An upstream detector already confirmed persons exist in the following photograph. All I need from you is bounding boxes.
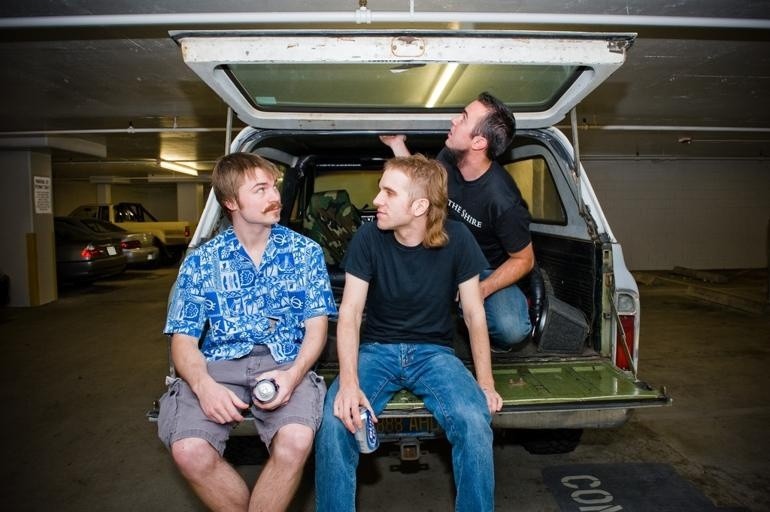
[314,153,503,512]
[380,92,535,353]
[156,152,339,512]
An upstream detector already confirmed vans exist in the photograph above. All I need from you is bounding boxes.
[141,20,676,461]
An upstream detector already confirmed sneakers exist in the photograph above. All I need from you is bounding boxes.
[490,345,514,354]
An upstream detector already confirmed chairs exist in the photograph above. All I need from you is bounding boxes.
[301,189,362,268]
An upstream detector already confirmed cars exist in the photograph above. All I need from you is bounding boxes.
[53,215,130,290]
[75,215,159,266]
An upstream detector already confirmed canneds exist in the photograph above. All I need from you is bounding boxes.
[350,405,381,455]
[250,378,279,403]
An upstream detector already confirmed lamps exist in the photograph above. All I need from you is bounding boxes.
[156,160,198,178]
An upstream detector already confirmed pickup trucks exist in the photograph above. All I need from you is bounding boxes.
[68,201,193,259]
